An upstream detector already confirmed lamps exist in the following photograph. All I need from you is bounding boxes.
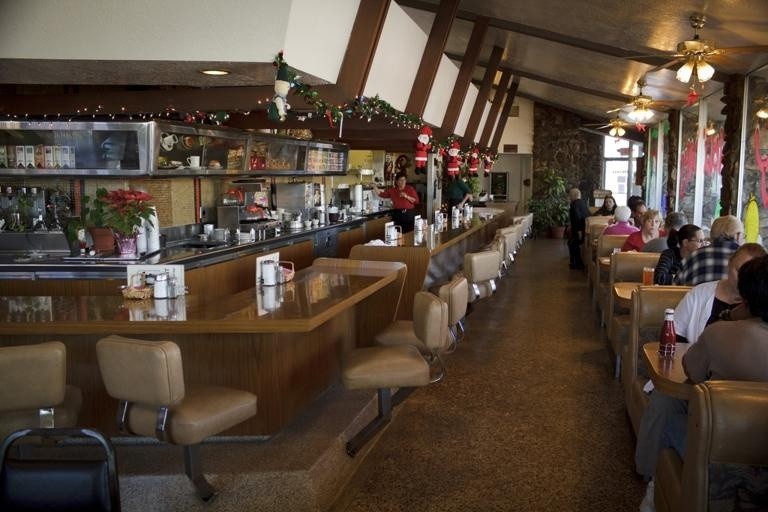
[627,109,655,121]
[676,59,715,83]
[609,127,626,137]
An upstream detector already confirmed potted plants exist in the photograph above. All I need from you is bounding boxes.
[542,173,571,239]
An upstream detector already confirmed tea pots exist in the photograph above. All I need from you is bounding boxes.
[223,188,242,206]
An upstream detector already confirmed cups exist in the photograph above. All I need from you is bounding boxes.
[163,134,178,146]
[305,220,311,227]
[185,135,194,147]
[312,219,319,225]
[186,154,200,169]
[329,207,338,224]
[135,205,159,254]
[642,265,655,285]
[613,247,621,253]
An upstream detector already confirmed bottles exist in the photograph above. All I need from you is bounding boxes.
[262,260,275,287]
[383,203,474,247]
[235,228,241,246]
[154,273,168,298]
[224,228,230,241]
[249,227,256,243]
[276,266,285,283]
[168,278,177,297]
[658,307,677,359]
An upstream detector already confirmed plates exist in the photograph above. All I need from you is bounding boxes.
[159,131,174,151]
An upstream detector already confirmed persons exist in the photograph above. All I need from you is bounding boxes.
[621,208,666,254]
[652,223,707,286]
[631,200,647,230]
[594,195,618,215]
[372,171,421,234]
[566,187,592,271]
[671,214,747,287]
[602,205,637,235]
[443,175,470,218]
[640,209,690,254]
[678,257,767,386]
[627,194,640,203]
[634,242,767,512]
[459,175,472,204]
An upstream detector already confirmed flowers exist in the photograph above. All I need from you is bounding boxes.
[100,189,156,234]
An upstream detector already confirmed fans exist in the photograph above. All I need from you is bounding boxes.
[606,79,688,114]
[623,14,768,73]
[583,115,647,129]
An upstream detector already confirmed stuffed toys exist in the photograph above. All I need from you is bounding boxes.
[466,146,482,179]
[446,141,461,181]
[266,65,294,123]
[482,150,496,178]
[413,124,434,175]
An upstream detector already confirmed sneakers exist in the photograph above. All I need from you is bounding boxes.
[638,480,655,512]
